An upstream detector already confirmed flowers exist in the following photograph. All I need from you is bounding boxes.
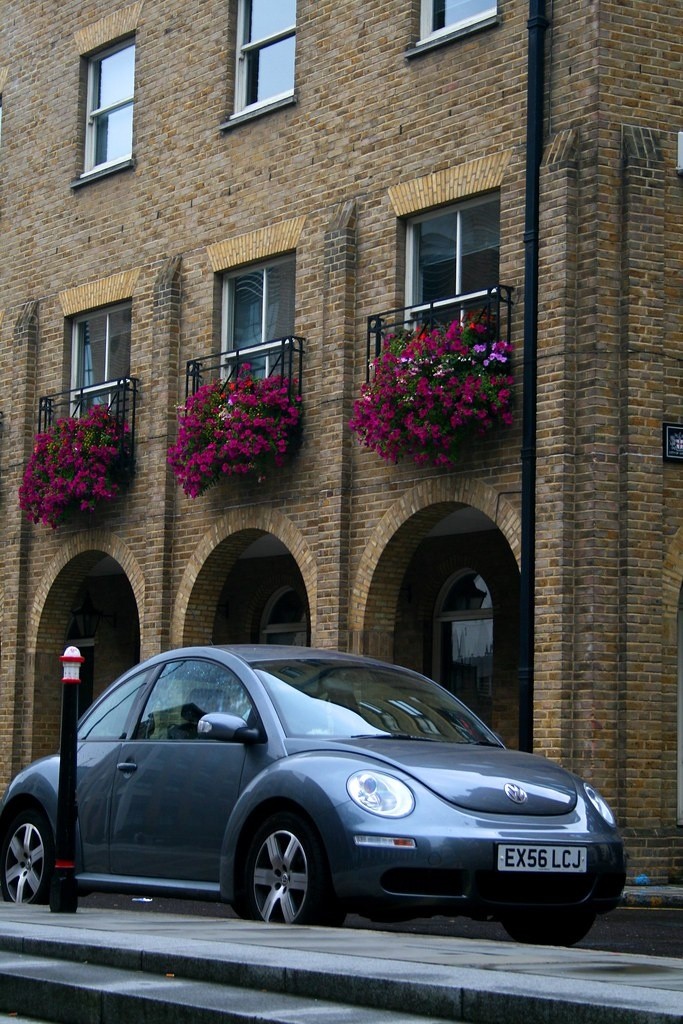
[165,360,303,498]
[346,306,516,467]
[17,402,132,533]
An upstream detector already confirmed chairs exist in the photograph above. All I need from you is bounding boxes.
[314,689,368,734]
[169,687,231,738]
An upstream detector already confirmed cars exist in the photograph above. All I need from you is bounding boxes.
[0,643,628,948]
